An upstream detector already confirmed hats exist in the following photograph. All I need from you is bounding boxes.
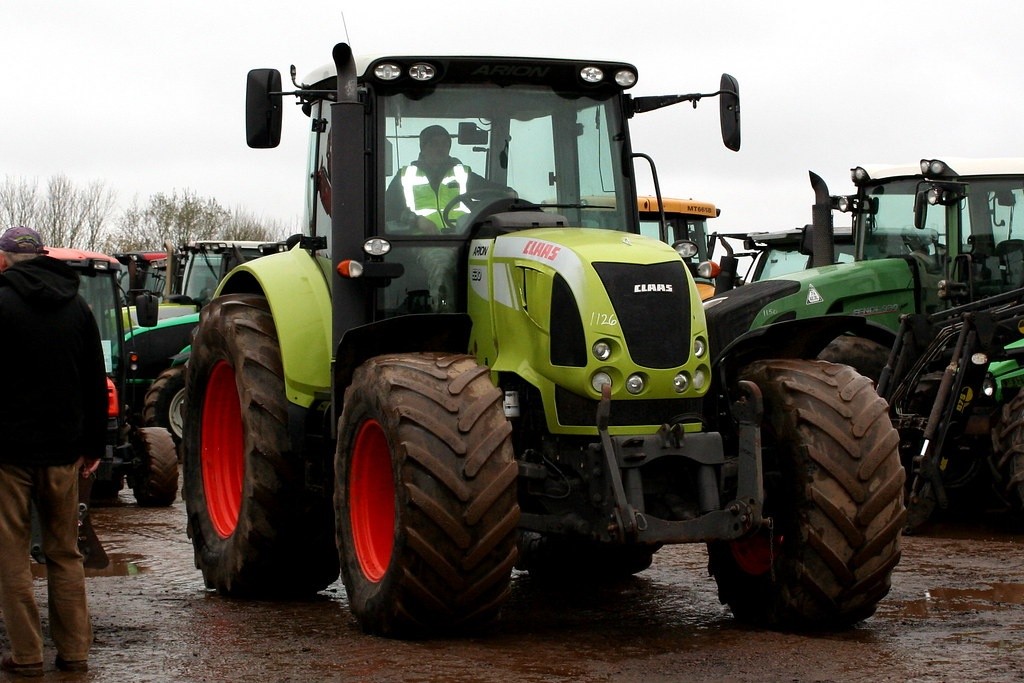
[0,226,49,254]
[420,125,452,150]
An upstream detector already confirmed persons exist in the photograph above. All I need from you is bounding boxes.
[384,125,520,236]
[0,227,109,676]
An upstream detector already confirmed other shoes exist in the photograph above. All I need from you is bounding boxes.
[0,654,43,676]
[55,653,88,673]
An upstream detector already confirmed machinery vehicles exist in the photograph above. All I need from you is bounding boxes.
[38,239,295,509]
[169,41,906,630]
[548,159,1024,518]
